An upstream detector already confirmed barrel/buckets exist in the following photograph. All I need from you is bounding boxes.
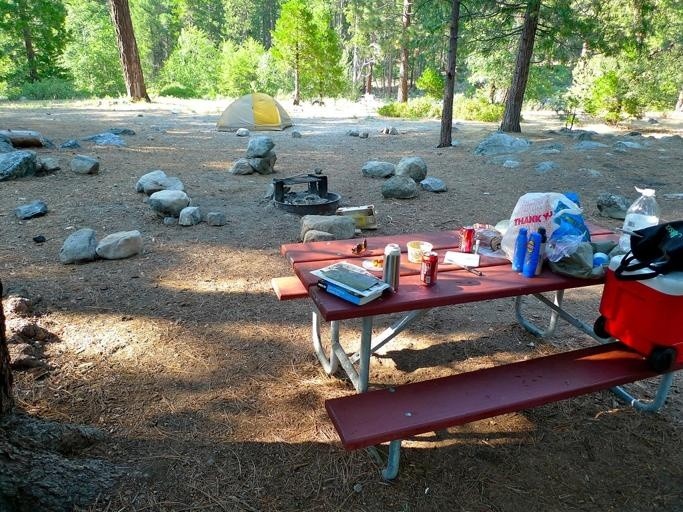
[617,185,662,254]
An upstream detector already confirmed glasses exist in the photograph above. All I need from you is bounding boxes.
[352,239,367,254]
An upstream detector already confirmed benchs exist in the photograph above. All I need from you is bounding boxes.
[269,274,570,376]
[324,339,670,451]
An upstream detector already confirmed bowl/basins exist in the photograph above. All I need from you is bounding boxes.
[406,240,433,264]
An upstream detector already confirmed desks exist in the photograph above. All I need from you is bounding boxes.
[281,220,622,322]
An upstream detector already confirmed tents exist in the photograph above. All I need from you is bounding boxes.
[217,92,293,131]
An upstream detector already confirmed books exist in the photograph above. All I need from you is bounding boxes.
[316,279,383,306]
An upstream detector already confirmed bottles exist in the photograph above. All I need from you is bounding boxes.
[522,231,542,279]
[535,226,547,276]
[381,243,400,292]
[512,227,528,272]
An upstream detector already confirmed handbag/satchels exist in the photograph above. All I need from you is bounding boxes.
[614,220,683,281]
[549,193,591,242]
[547,240,627,280]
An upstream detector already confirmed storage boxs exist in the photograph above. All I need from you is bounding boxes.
[594,253,683,372]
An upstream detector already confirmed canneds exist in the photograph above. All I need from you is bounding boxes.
[422,251,438,287]
[459,227,475,254]
[490,236,502,252]
